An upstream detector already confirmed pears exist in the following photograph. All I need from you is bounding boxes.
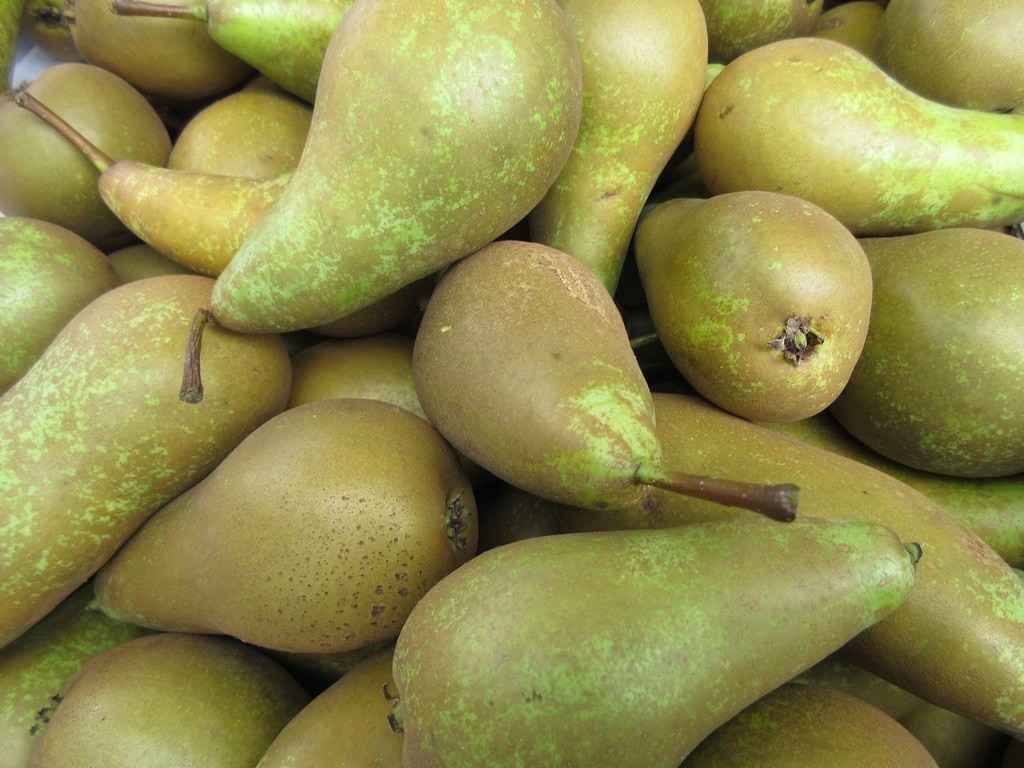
[1,0,1024,768]
